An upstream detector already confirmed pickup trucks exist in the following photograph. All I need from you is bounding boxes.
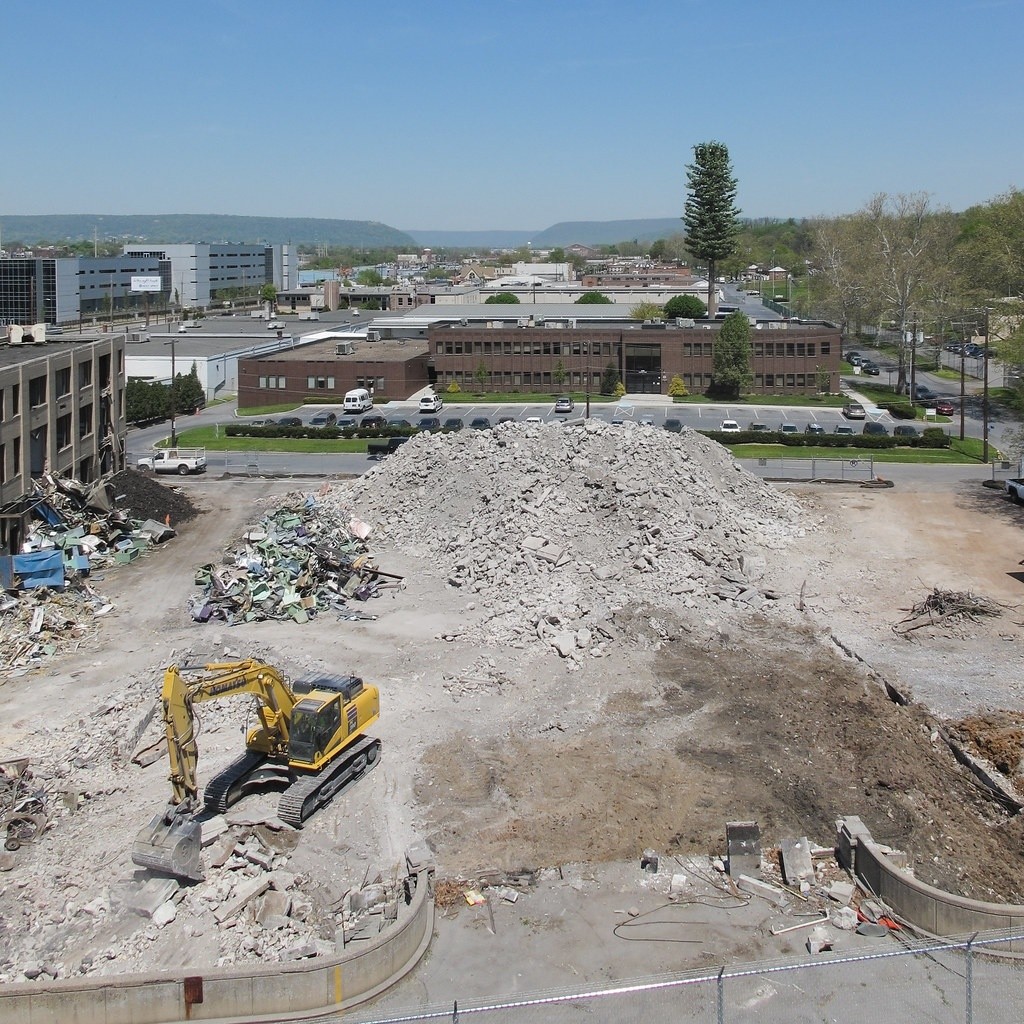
[368,438,410,460]
[1006,478,1024,503]
[136,447,208,475]
[277,417,302,428]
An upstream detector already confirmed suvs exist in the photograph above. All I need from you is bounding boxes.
[719,419,742,433]
[309,411,338,429]
[778,423,801,434]
[419,396,443,413]
[804,423,827,435]
[360,416,387,430]
[863,422,889,438]
[749,423,771,434]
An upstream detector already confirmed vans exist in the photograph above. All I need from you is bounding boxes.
[343,388,373,414]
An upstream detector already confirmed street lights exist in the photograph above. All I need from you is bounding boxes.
[164,338,180,448]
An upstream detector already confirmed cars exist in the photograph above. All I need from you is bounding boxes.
[442,418,463,430]
[944,341,1000,357]
[525,417,544,425]
[894,426,920,439]
[555,398,575,413]
[834,425,856,437]
[469,417,491,428]
[844,351,879,375]
[611,419,623,427]
[936,400,953,416]
[495,417,517,424]
[251,418,276,428]
[553,417,567,422]
[662,419,684,433]
[640,419,655,427]
[388,420,411,430]
[906,382,940,408]
[415,417,441,429]
[336,418,359,431]
[843,403,866,419]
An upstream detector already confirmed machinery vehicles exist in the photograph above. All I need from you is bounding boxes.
[129,657,381,881]
